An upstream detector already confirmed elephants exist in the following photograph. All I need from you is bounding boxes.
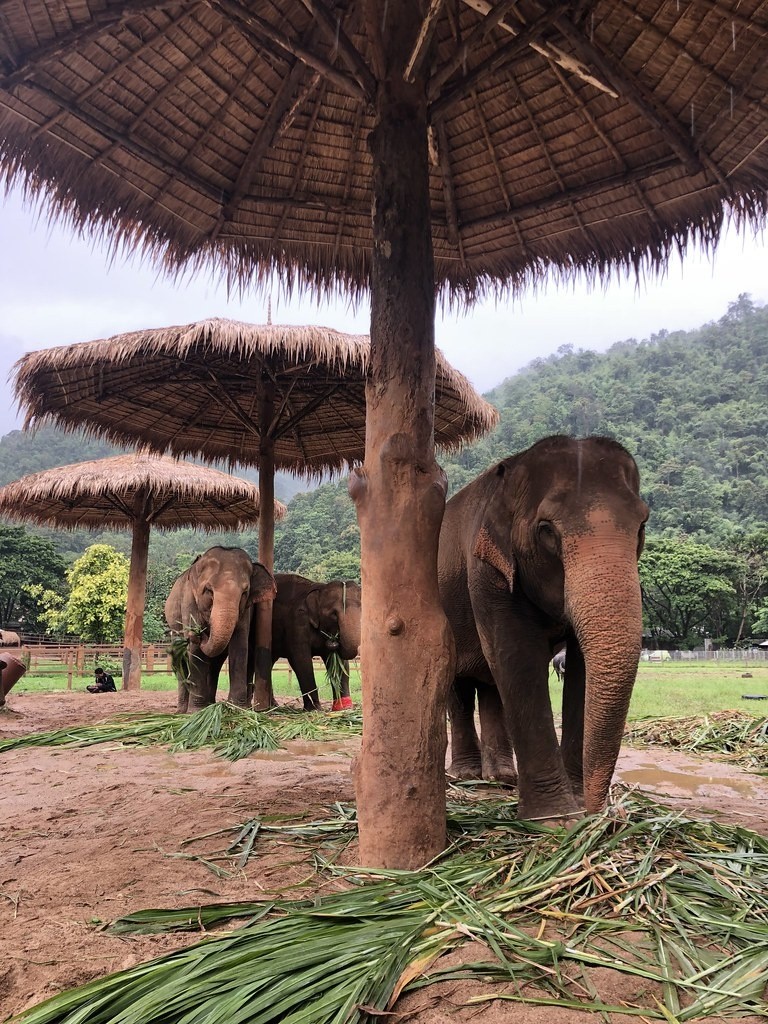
[434,434,649,831]
[164,545,363,717]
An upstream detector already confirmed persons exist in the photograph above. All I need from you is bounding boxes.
[87,668,117,694]
